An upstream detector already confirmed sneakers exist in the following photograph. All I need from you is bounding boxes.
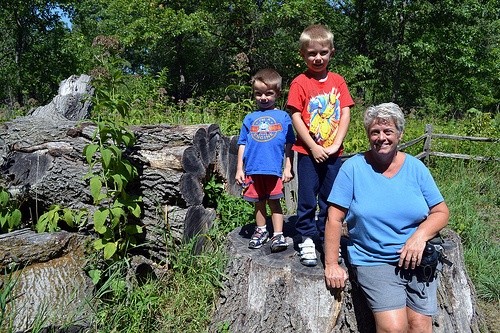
[298,239,317,265]
[271,234,289,253]
[248,228,270,249]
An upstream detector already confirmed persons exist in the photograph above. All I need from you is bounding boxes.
[287,25,355,266]
[235,69,295,253]
[324,102,450,333]
